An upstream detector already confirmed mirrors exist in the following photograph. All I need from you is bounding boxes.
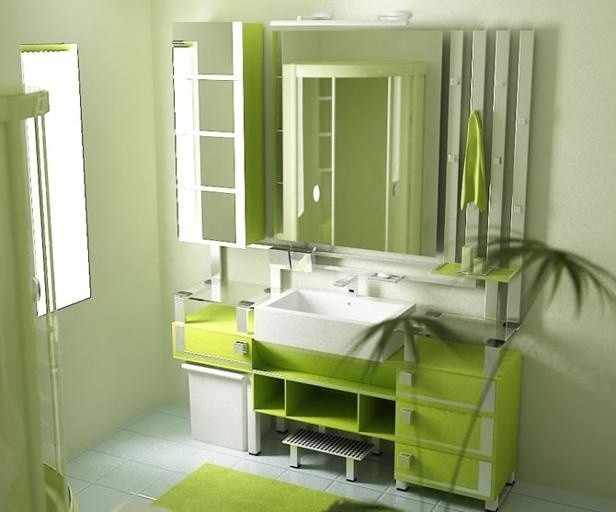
[270,28,443,262]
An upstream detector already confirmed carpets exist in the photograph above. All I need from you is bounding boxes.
[150,460,399,512]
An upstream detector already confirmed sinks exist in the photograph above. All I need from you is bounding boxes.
[255,289,417,362]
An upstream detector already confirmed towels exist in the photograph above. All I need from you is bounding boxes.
[458,109,491,214]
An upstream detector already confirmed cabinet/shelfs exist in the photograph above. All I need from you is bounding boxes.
[171,274,291,371]
[252,340,523,512]
[171,19,265,247]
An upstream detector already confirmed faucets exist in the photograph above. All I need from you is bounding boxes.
[332,274,358,295]
